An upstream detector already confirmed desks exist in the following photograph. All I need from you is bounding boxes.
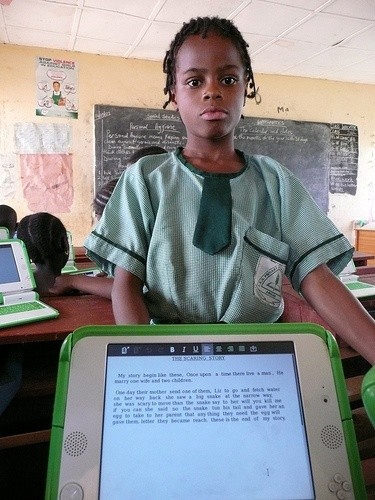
[0,281,374,451]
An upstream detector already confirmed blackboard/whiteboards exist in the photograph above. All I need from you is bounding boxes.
[93,104,330,220]
[330,123,358,196]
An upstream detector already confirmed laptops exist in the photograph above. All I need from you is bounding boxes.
[43,323,368,500]
[336,257,375,301]
[0,238,60,329]
[0,226,102,277]
[360,364,375,429]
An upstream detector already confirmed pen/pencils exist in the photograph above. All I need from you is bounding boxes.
[74,271,93,277]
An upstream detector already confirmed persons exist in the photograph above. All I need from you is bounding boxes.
[0,147,168,299]
[51,81,63,106]
[85,18,375,367]
